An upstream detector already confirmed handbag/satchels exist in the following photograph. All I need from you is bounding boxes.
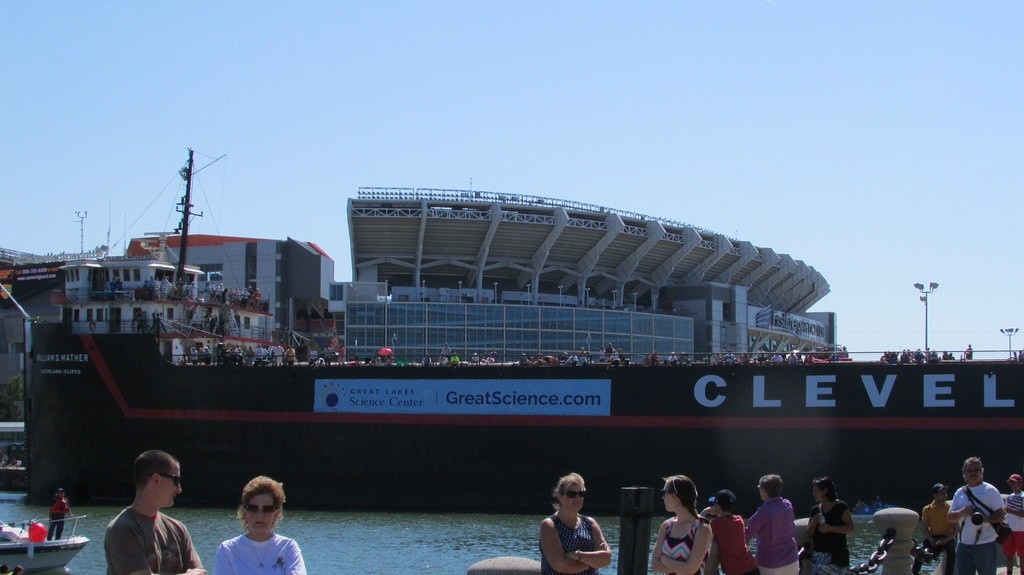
[991,520,1012,545]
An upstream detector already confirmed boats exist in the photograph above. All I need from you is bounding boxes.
[0,514,91,575]
[23,149,1024,518]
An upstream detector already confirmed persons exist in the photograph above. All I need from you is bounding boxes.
[965,345,973,360]
[190,338,295,367]
[104,449,207,575]
[207,280,262,307]
[947,456,1005,575]
[539,473,612,575]
[652,476,714,575]
[1001,473,1024,575]
[911,482,960,575]
[699,489,760,575]
[303,343,692,368]
[855,494,885,510]
[109,278,123,300]
[880,348,954,363]
[0,562,25,575]
[808,476,856,575]
[204,312,218,333]
[216,475,307,575]
[746,474,800,575]
[143,274,196,301]
[130,308,149,335]
[704,346,838,368]
[46,488,74,541]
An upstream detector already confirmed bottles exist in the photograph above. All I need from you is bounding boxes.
[818,512,825,528]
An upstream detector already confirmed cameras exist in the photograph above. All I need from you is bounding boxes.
[971,508,985,525]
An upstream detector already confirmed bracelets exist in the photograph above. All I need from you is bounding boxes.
[576,550,581,562]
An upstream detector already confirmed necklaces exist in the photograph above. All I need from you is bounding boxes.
[248,533,273,567]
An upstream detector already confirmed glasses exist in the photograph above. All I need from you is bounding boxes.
[244,504,279,513]
[566,491,588,498]
[145,472,181,486]
[660,488,674,497]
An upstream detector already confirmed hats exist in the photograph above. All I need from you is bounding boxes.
[708,490,737,505]
[56,488,63,493]
[931,483,948,494]
[1006,474,1022,486]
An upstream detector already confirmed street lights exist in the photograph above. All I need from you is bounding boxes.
[1000,328,1019,358]
[913,281,939,350]
